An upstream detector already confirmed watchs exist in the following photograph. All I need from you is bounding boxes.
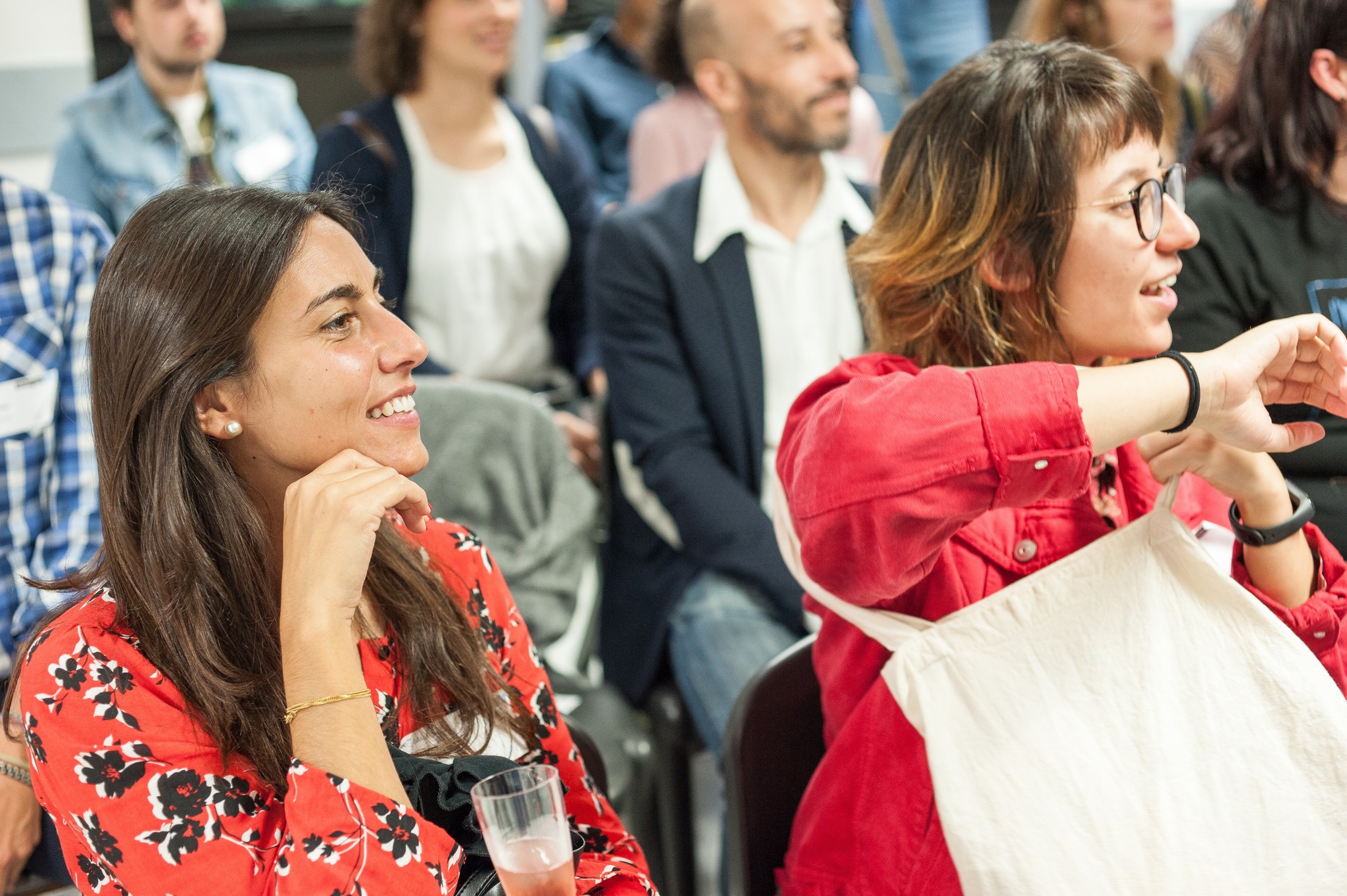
[1226,478,1316,548]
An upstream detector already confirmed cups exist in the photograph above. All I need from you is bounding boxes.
[469,764,577,896]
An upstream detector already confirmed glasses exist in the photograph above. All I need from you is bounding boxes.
[1038,162,1189,244]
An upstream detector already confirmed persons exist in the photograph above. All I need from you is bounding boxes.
[538,1,668,209]
[54,0,318,235]
[628,0,882,205]
[599,2,877,896]
[0,183,129,896]
[0,185,661,896]
[774,38,1347,895]
[1181,0,1347,555]
[308,0,607,484]
[1014,1,1212,150]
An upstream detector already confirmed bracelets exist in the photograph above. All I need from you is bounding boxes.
[1153,350,1201,433]
[0,710,25,726]
[284,687,372,723]
[0,755,29,771]
[0,764,33,788]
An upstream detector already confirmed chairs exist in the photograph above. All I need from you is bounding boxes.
[406,377,822,896]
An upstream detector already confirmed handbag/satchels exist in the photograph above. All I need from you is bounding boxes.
[774,472,1347,896]
[454,828,585,895]
